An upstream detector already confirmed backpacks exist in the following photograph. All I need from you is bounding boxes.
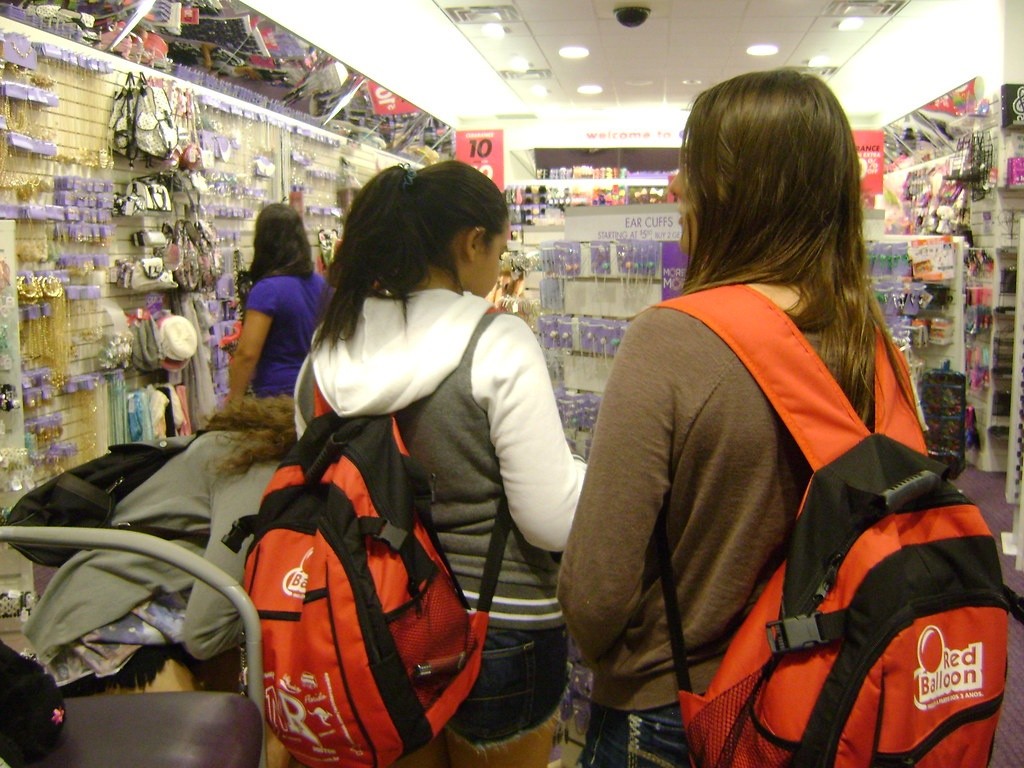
[653,283,1009,768]
[223,384,511,768]
[108,74,216,174]
[5,429,210,568]
[36,5,99,46]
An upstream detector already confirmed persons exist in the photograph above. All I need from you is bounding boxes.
[25,395,299,695]
[294,161,587,768]
[558,68,913,768]
[226,204,325,404]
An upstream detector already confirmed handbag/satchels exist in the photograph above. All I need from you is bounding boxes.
[115,171,221,295]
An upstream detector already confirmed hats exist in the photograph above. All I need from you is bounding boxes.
[132,312,200,372]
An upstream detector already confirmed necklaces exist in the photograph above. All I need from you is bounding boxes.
[0,35,117,480]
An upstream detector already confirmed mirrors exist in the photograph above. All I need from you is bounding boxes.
[0,0,455,166]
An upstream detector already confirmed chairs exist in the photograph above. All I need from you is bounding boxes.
[0,519,269,768]
